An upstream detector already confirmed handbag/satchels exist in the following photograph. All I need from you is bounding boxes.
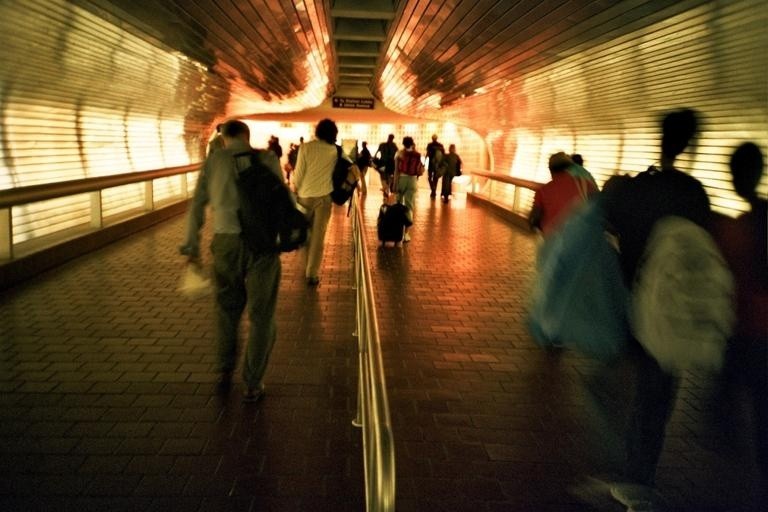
[331,145,360,206]
[416,161,424,176]
[634,215,733,372]
[232,149,306,256]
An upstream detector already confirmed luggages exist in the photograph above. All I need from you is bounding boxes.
[377,192,407,245]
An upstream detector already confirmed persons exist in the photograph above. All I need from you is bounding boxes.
[173,117,310,406]
[590,108,711,508]
[345,134,463,248]
[569,152,601,202]
[521,151,590,363]
[703,140,766,484]
[205,124,303,184]
[289,118,352,286]
